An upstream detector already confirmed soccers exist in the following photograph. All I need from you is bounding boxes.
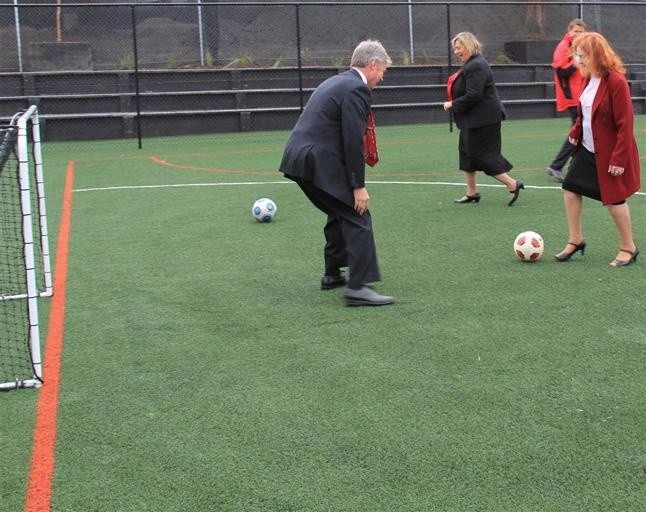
[514,231,544,261]
[252,198,277,223]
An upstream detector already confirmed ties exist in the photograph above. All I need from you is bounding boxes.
[360,104,381,170]
[447,66,463,99]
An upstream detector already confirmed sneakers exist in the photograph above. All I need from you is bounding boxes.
[544,166,564,181]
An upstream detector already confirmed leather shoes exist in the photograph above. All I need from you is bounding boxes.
[319,273,349,292]
[341,284,396,308]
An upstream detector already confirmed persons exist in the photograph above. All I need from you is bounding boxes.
[442,31,524,206]
[555,32,642,267]
[545,18,586,183]
[279,39,396,306]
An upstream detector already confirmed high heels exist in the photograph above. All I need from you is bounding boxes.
[452,191,481,203]
[506,179,525,206]
[553,236,587,261]
[607,244,639,267]
